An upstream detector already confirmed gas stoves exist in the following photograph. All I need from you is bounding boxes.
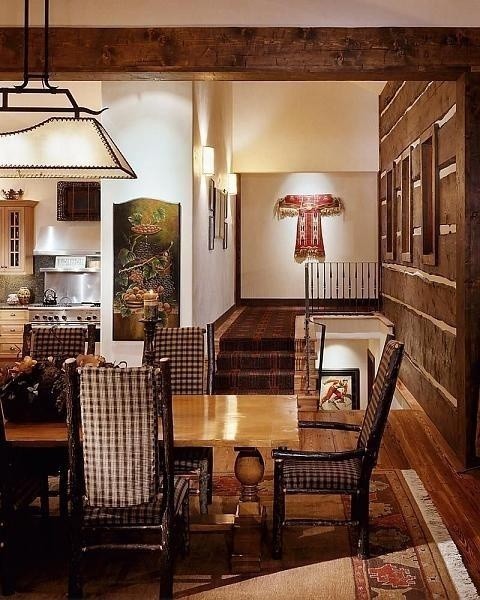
[28,303,101,321]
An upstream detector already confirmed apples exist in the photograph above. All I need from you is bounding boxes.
[122,287,146,300]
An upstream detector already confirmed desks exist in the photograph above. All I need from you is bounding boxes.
[0,382,304,576]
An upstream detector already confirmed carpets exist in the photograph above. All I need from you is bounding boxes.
[0,461,480,600]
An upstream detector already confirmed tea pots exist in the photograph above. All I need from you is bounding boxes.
[43,288,57,305]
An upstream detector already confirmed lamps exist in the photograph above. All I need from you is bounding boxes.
[227,170,239,196]
[0,0,139,189]
[200,144,218,178]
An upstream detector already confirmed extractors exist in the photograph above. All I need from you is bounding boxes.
[31,224,101,256]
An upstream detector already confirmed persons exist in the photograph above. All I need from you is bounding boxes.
[319,379,352,407]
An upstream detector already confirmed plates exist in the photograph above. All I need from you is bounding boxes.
[130,223,163,235]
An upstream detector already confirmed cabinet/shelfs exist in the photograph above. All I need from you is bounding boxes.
[1,309,33,360]
[56,181,100,223]
[0,197,39,275]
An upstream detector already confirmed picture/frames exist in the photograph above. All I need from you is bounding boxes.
[316,366,362,411]
[207,177,228,254]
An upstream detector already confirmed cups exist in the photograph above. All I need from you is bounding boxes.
[142,293,160,322]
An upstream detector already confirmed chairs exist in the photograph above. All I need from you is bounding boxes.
[267,330,409,562]
[142,312,217,514]
[58,350,193,599]
[0,400,51,596]
[14,323,100,518]
